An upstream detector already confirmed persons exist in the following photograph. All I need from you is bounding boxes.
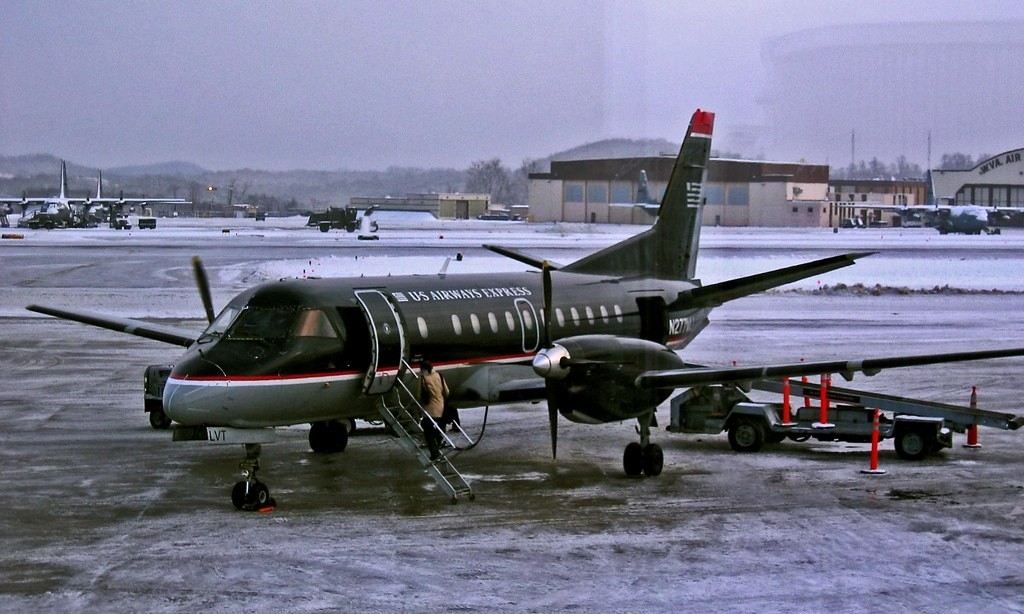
[417,359,450,460]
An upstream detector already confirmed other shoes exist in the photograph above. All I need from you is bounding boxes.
[429,457,441,462]
[437,438,446,449]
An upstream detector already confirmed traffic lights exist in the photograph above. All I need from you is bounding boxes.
[206,186,217,191]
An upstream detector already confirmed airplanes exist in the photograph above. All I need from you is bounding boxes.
[1,158,193,232]
[25,107,1024,515]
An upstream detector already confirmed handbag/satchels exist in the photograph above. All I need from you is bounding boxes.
[443,400,454,424]
[420,375,430,405]
